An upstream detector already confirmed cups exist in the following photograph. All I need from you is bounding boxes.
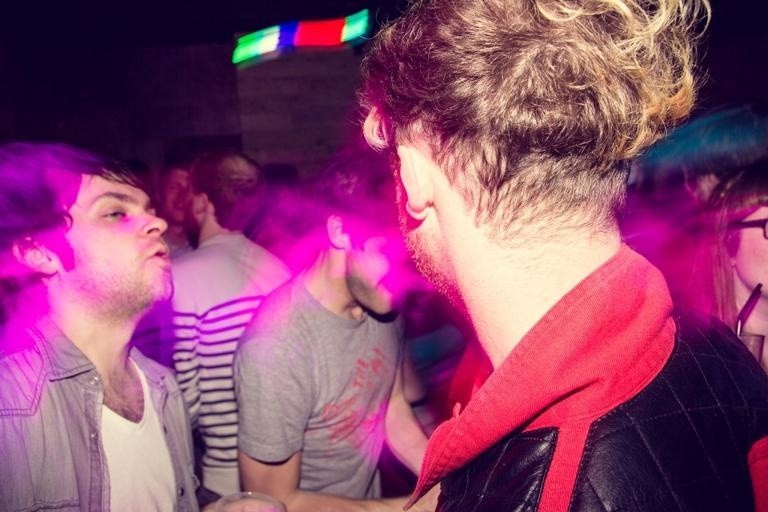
[737,330,764,364]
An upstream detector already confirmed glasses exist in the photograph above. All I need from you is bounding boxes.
[730,218,767,239]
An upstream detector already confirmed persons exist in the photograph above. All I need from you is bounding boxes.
[360,2,766,511]
[0,143,441,510]
